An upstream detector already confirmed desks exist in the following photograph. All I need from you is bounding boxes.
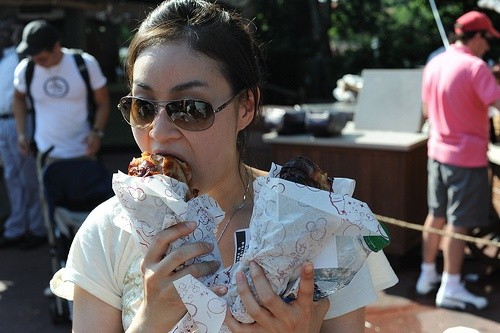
[261,129,429,268]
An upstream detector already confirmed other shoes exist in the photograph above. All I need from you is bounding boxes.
[20,235,47,252]
[0,238,22,249]
[436,290,488,310]
[416,274,441,295]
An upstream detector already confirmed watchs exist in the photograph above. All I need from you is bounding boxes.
[90,127,105,137]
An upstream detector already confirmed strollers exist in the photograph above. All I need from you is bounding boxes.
[37,137,119,326]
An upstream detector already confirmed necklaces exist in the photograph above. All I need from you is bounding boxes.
[217,168,251,245]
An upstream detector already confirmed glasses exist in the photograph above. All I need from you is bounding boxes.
[117,88,249,132]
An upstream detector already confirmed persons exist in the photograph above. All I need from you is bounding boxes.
[50,0,399,333]
[136,99,152,119]
[417,10,500,312]
[186,101,203,118]
[13,21,108,295]
[0,17,49,243]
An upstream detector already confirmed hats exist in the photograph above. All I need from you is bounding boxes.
[17,20,56,56]
[456,11,499,38]
